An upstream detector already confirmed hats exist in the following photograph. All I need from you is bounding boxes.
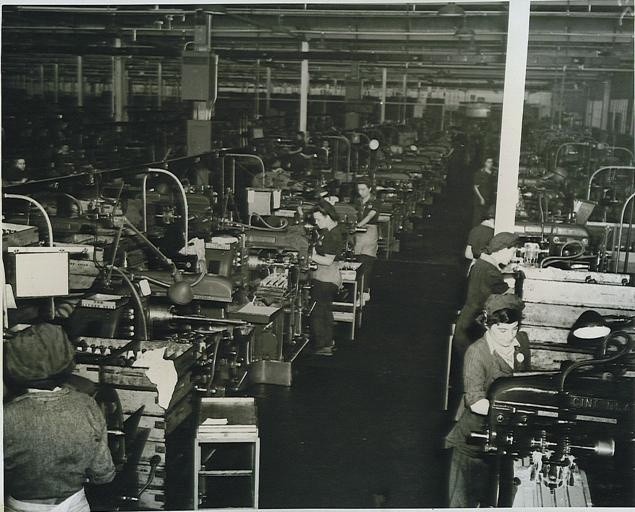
[319,198,335,212]
[482,294,525,315]
[484,232,520,254]
[2,323,77,391]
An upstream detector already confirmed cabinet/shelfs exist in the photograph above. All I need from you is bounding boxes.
[193,396,260,509]
[331,262,366,341]
[378,213,395,260]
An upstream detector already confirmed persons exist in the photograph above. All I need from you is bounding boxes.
[312,140,332,172]
[442,290,533,508]
[3,323,117,512]
[469,156,495,235]
[7,156,36,196]
[446,230,523,416]
[464,203,498,293]
[287,130,306,174]
[304,198,348,358]
[328,173,382,295]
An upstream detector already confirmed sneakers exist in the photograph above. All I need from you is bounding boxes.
[311,339,337,359]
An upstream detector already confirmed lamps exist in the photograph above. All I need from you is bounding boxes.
[107,217,193,306]
[434,1,475,40]
[573,310,612,341]
